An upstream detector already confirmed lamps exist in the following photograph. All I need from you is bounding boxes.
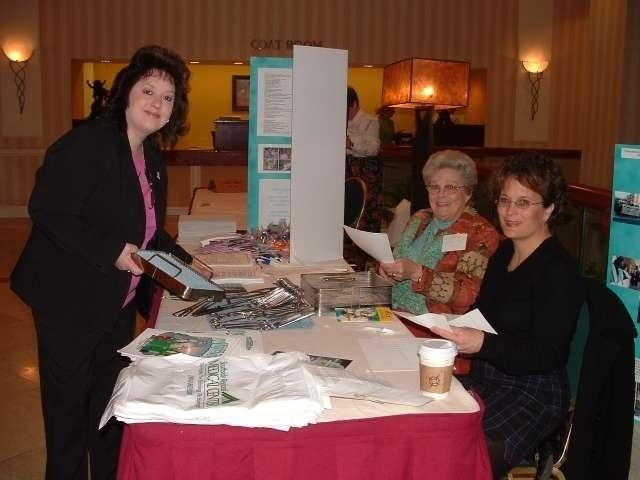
[382,58,468,209]
[521,58,550,120]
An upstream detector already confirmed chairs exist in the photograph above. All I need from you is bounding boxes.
[506,281,633,480]
[345,174,367,247]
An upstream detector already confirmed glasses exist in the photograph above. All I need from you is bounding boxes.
[425,184,468,194]
[138,169,154,209]
[494,197,544,209]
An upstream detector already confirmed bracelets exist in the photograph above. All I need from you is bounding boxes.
[412,278,422,283]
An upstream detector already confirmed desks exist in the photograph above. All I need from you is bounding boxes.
[118,186,485,478]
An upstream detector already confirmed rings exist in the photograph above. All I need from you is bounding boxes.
[127,269,131,273]
[392,272,397,278]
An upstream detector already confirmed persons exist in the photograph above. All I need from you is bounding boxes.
[346,86,380,173]
[378,148,501,339]
[31,45,190,479]
[431,155,570,478]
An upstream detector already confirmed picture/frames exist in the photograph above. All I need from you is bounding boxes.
[232,74,249,111]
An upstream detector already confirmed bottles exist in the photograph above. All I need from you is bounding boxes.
[417,339,458,400]
